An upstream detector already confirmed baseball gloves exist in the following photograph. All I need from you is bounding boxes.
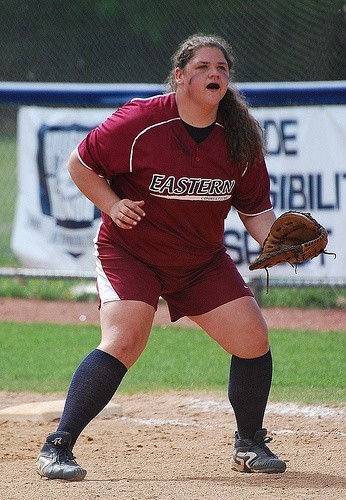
[249,210,336,295]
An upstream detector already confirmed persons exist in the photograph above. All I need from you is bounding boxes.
[34,32,329,480]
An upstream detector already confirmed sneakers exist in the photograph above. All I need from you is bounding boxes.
[34,430,86,483]
[228,428,286,474]
